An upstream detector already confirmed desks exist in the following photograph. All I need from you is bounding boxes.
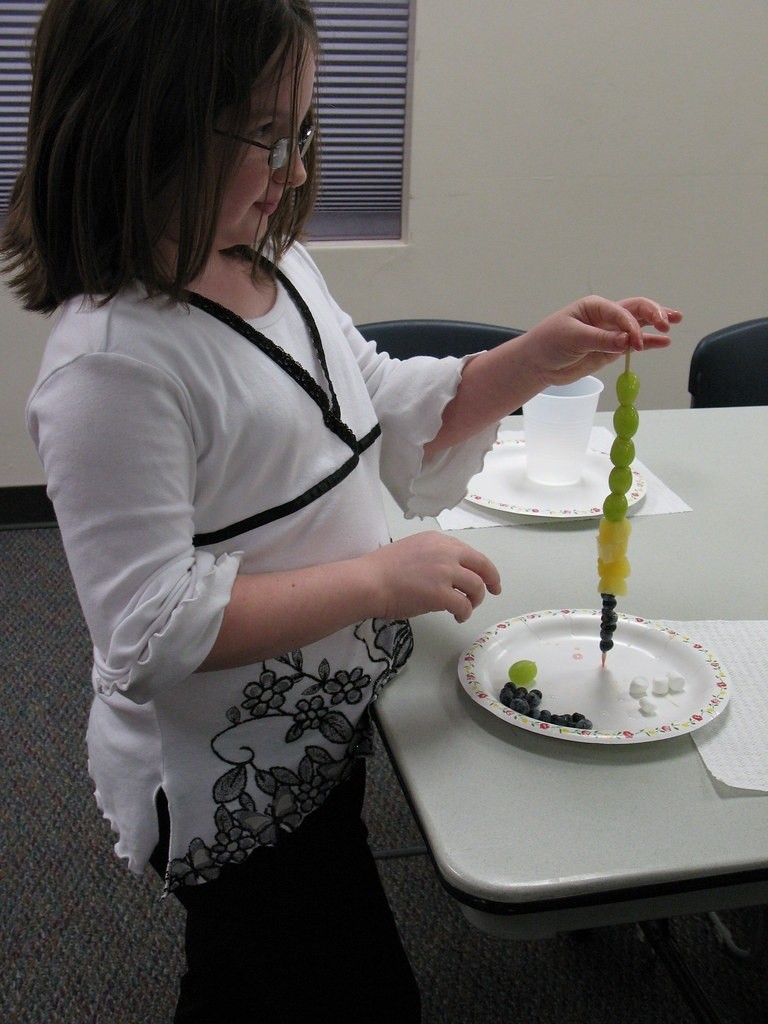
[370,405,768,1023]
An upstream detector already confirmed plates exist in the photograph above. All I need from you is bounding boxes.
[454,607,732,745]
[461,439,649,520]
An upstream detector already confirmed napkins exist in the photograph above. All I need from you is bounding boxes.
[650,620,768,794]
[434,426,694,532]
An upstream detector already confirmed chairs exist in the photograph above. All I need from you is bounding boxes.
[352,320,526,415]
[687,317,768,408]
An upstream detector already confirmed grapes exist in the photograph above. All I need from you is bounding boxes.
[603,372,640,521]
[509,660,537,684]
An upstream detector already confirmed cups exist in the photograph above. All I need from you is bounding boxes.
[519,375,605,485]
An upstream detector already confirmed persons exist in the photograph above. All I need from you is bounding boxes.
[1,0,682,1023]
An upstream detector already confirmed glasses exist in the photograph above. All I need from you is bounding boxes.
[203,124,315,171]
